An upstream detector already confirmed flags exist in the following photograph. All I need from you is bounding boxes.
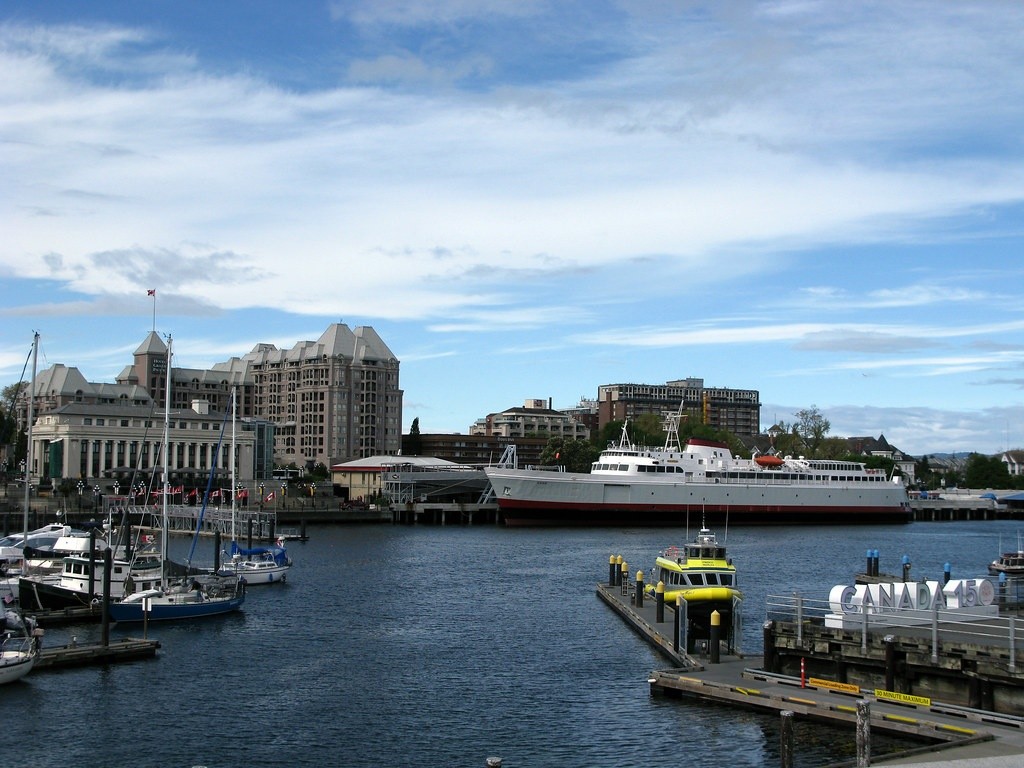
[4,593,14,603]
[552,452,561,459]
[138,485,276,503]
[148,290,155,296]
[141,535,155,543]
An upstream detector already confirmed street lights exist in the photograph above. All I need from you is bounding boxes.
[113,480,121,496]
[311,482,317,510]
[93,484,102,513]
[258,482,266,512]
[281,482,288,510]
[236,482,244,509]
[76,479,85,520]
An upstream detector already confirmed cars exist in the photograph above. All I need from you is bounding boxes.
[340,499,370,512]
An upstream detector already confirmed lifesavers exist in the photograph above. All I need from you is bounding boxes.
[668,545,679,557]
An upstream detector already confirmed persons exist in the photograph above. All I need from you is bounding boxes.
[649,588,655,597]
[189,577,204,602]
[357,496,362,501]
[123,573,133,597]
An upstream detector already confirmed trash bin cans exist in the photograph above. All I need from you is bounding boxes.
[699,641,707,658]
[630,592,635,605]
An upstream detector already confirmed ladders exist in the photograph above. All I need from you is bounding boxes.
[622,577,628,596]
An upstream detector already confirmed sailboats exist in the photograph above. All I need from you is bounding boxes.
[0,328,174,622]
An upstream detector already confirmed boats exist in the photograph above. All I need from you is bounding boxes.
[649,498,744,640]
[0,633,38,684]
[483,398,915,530]
[987,528,1024,576]
[168,386,293,587]
[109,334,249,623]
